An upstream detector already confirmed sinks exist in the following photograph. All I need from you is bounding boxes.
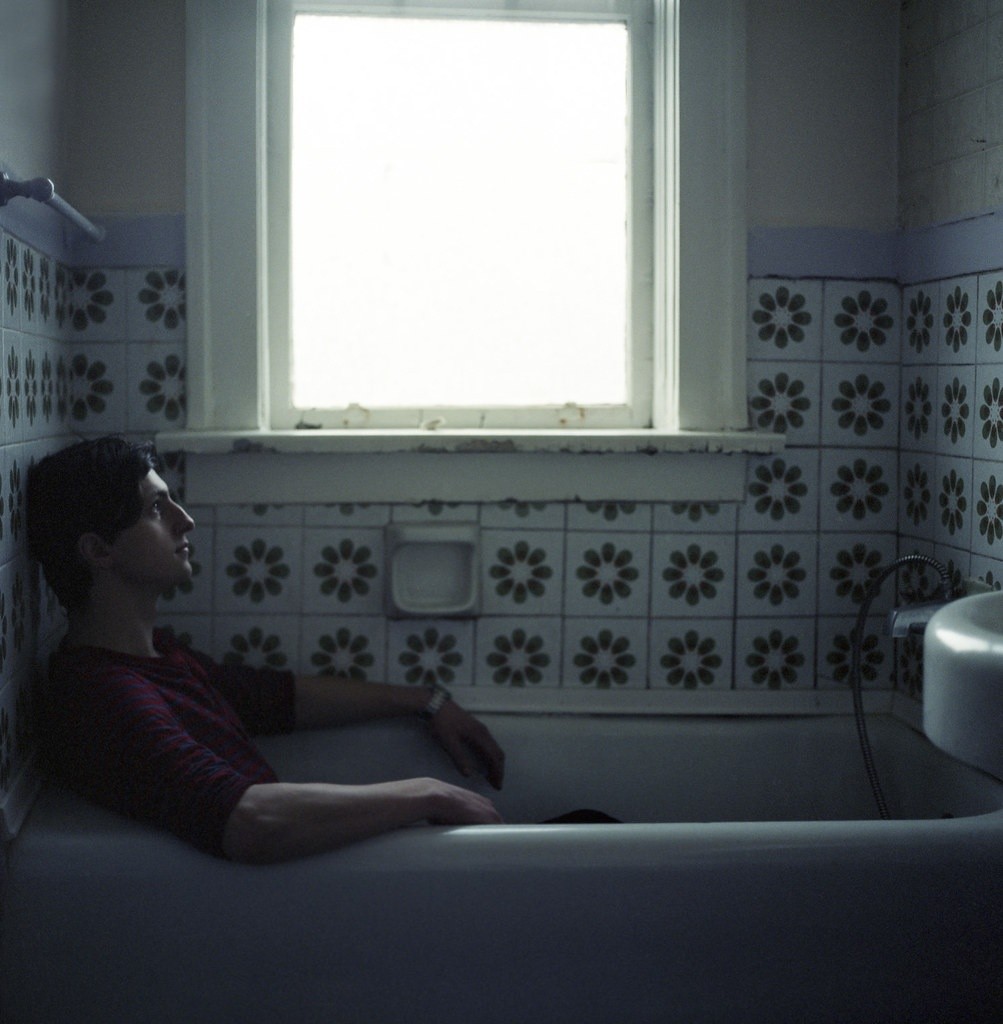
[921,588,1003,775]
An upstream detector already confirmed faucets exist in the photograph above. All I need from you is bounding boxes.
[886,597,957,639]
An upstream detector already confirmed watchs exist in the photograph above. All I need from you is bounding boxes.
[425,688,450,716]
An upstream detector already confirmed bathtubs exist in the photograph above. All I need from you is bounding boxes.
[0,710,1003,1024]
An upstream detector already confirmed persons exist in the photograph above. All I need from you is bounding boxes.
[28,435,619,863]
